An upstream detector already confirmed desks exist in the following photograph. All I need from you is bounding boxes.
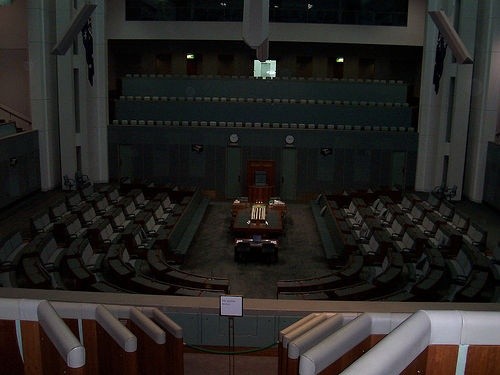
[234,238,279,265]
[234,207,282,235]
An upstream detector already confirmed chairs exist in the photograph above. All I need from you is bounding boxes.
[235,241,277,266]
[1,297,187,375]
[277,310,500,375]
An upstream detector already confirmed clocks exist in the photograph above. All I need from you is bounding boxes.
[285,134,294,144]
[230,133,238,143]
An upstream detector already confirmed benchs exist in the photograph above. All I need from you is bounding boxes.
[0,171,231,296]
[275,182,500,303]
